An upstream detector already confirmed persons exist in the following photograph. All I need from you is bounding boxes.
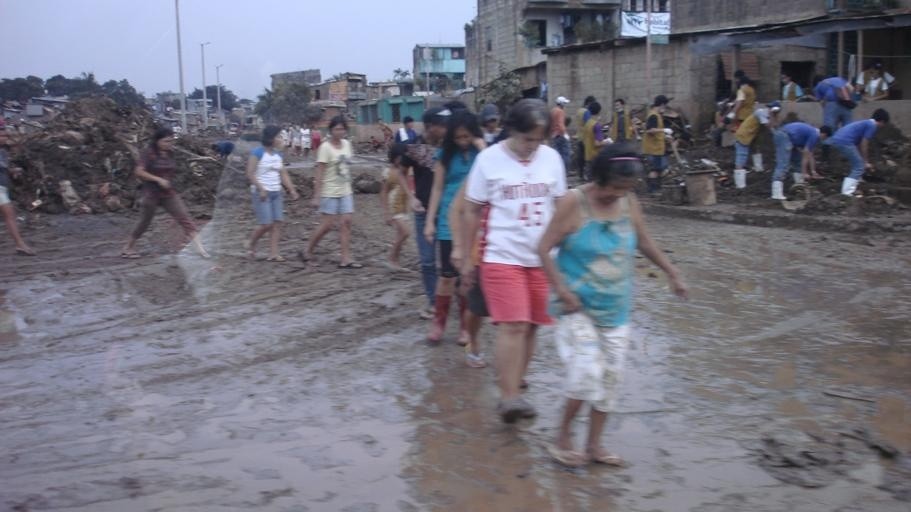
[714,100,727,127]
[780,72,806,101]
[243,125,300,262]
[301,116,367,268]
[210,140,234,164]
[733,101,782,191]
[447,124,510,370]
[540,79,548,101]
[377,118,394,151]
[709,103,733,146]
[462,98,567,423]
[639,95,675,199]
[371,136,381,150]
[821,107,890,200]
[394,116,419,178]
[610,99,642,143]
[379,144,415,274]
[422,109,488,345]
[281,123,321,158]
[480,104,505,144]
[121,129,211,260]
[407,105,462,319]
[583,101,614,182]
[549,94,574,189]
[576,94,597,181]
[864,65,889,101]
[537,146,689,466]
[770,123,834,201]
[855,70,896,93]
[723,66,757,128]
[811,74,871,162]
[1,114,36,255]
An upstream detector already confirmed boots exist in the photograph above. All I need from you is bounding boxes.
[457,295,472,344]
[430,296,449,341]
[752,154,764,171]
[842,177,857,194]
[771,181,787,200]
[733,170,746,188]
[792,173,806,183]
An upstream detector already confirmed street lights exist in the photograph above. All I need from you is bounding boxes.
[199,41,210,129]
[215,64,225,108]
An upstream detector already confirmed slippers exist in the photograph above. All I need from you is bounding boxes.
[338,262,362,268]
[592,455,626,468]
[420,308,434,320]
[466,344,485,367]
[547,444,581,466]
[266,256,285,262]
[298,249,320,267]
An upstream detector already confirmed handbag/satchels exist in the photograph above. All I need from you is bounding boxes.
[836,86,857,109]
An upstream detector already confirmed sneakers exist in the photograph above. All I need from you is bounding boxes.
[497,399,523,419]
[523,398,537,416]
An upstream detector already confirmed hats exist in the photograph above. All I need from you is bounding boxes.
[483,104,500,122]
[655,95,673,105]
[555,96,570,104]
[423,107,452,122]
[767,102,780,107]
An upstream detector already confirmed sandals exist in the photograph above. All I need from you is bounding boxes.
[196,250,210,258]
[122,252,140,258]
